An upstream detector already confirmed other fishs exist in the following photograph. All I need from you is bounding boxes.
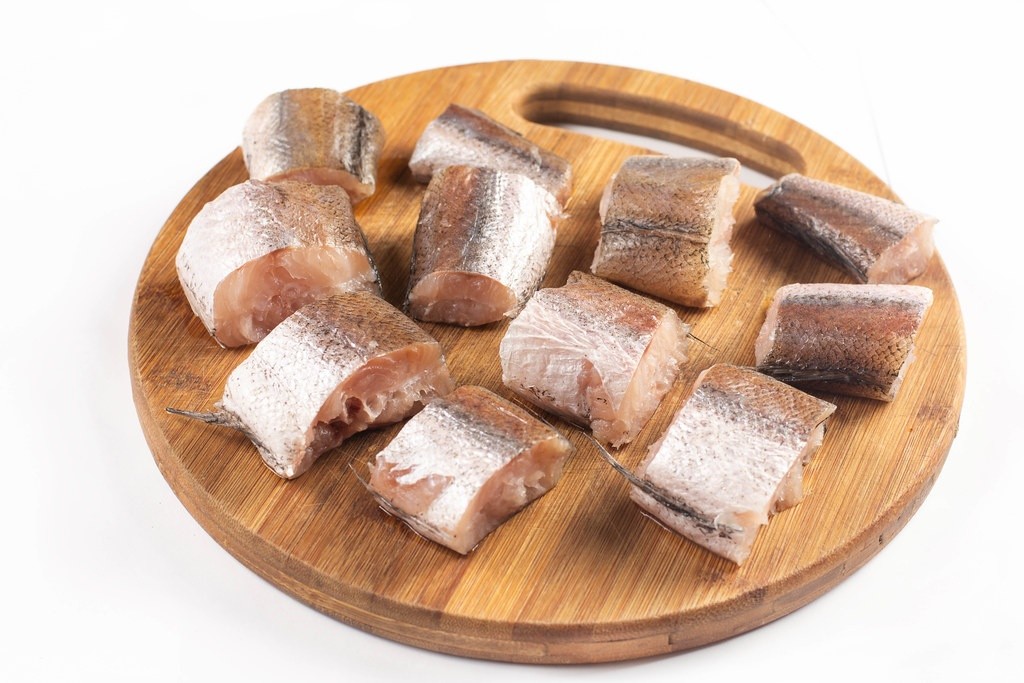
[173,87,948,568]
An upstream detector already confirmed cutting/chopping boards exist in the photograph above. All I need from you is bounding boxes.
[127,58,968,666]
